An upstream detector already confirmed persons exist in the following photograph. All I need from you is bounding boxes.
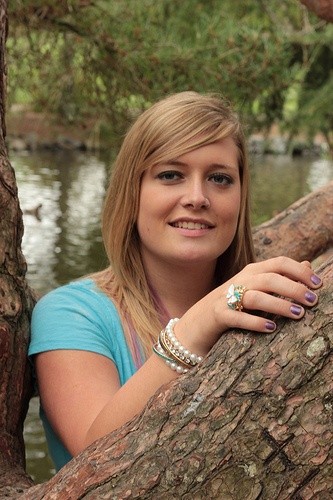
[28,90,324,474]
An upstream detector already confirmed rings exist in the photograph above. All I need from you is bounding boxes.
[225,282,247,311]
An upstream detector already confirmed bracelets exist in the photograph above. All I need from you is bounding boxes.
[151,317,205,374]
